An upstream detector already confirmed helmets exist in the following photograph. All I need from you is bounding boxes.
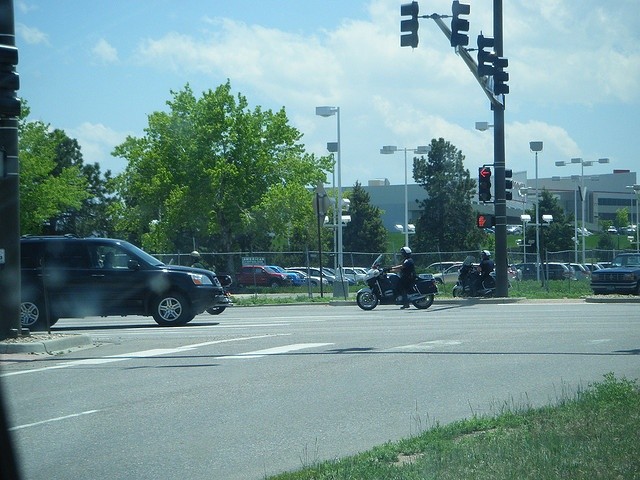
[190,251,200,257]
[481,249,492,258]
[400,247,412,257]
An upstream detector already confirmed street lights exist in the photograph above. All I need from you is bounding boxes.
[380,146,432,246]
[314,100,349,298]
[515,178,532,262]
[551,175,600,262]
[529,140,543,287]
[626,184,640,253]
[554,158,609,263]
[326,142,338,266]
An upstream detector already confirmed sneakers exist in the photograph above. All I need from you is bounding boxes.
[400,305,411,309]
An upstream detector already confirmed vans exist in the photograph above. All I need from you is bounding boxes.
[336,267,369,282]
[290,266,335,283]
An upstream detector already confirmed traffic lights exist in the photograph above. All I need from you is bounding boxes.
[479,168,490,202]
[504,169,512,202]
[452,0,471,50]
[478,31,495,79]
[494,56,510,97]
[478,215,493,229]
[400,2,419,50]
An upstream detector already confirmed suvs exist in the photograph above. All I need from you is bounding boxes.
[20,233,235,332]
[264,266,302,288]
[218,265,291,289]
[285,266,338,284]
[432,264,498,285]
[591,261,618,268]
[590,253,640,295]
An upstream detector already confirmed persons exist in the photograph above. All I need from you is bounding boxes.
[385,247,415,310]
[103,252,114,269]
[473,250,493,290]
[190,250,205,269]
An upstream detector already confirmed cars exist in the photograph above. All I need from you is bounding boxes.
[586,263,603,269]
[540,264,568,280]
[597,261,619,268]
[319,267,355,286]
[284,269,329,289]
[571,264,590,275]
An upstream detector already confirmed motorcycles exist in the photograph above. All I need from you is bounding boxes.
[452,256,513,299]
[356,254,439,311]
[167,258,234,316]
